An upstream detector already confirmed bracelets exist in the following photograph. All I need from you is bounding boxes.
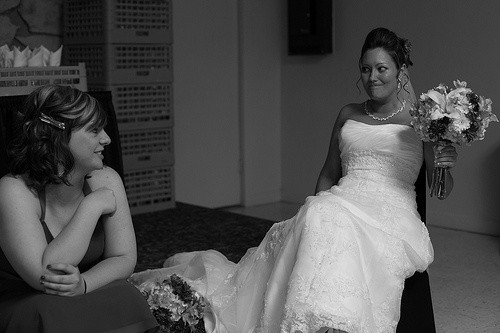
[83,279,87,294]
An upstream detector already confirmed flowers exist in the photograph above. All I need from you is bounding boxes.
[142,275,206,332]
[409,79,499,199]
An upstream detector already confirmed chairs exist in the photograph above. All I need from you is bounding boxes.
[397,160,437,332]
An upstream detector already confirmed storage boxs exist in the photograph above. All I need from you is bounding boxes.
[62,0,174,44]
[117,170,175,214]
[62,44,173,82]
[88,84,173,129]
[0,63,89,97]
[102,130,175,171]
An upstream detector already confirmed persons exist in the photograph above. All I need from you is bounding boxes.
[299,28,457,333]
[0,83,160,333]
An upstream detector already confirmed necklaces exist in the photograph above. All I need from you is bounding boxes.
[364,99,406,121]
